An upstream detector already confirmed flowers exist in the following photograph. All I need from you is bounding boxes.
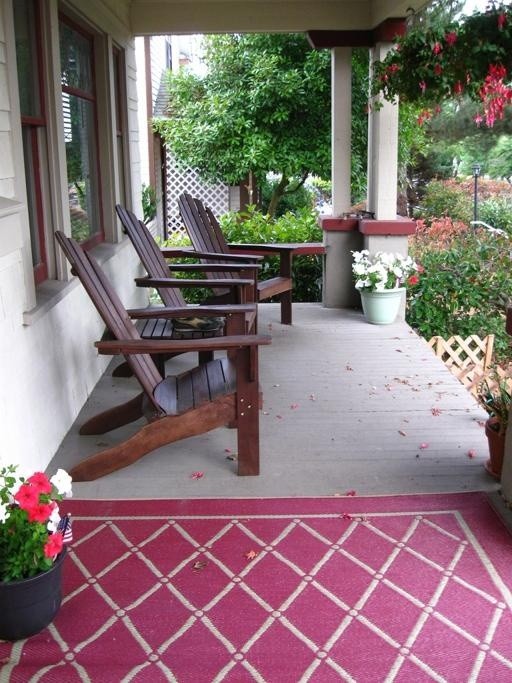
[1,464,73,582]
[472,364,512,435]
[350,249,420,295]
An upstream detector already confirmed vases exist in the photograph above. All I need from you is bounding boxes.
[0,542,69,642]
[359,286,406,325]
[485,417,508,476]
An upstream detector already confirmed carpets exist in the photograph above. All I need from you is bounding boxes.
[0,489,512,681]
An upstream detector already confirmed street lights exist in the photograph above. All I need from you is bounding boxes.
[471,161,482,231]
